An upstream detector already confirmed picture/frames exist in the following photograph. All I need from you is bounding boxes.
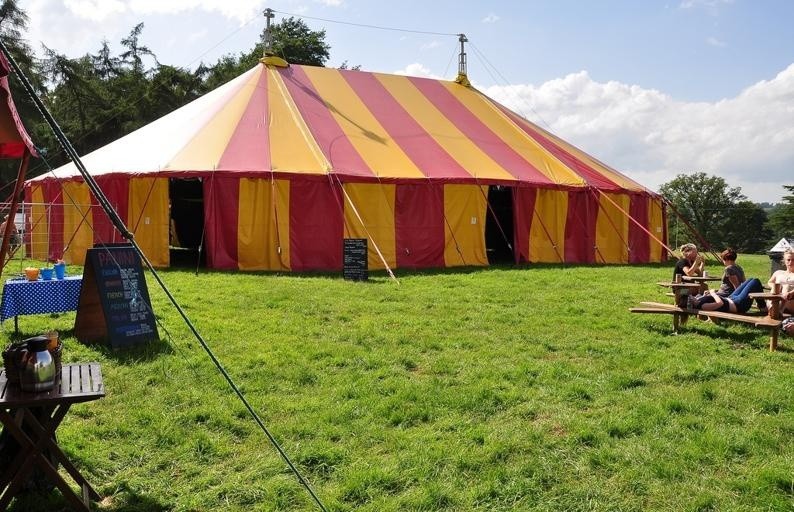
[749,283,786,352]
[4,275,83,335]
[656,276,721,336]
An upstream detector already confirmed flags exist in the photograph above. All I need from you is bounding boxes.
[25,263,65,281]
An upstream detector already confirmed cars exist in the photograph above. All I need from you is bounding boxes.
[675,273,683,284]
[24,262,67,282]
[770,283,781,295]
[703,270,709,278]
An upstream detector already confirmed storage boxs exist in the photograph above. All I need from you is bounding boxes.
[628,293,792,352]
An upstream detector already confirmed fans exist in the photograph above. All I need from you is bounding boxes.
[74,249,106,340]
[343,239,368,279]
[88,247,160,352]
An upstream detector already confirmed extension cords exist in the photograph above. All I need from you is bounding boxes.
[10,336,59,394]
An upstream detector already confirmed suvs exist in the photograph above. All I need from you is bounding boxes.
[40,269,53,280]
[24,270,38,281]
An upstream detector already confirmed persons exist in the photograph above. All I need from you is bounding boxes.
[715,247,746,296]
[679,278,769,317]
[671,242,705,296]
[766,247,793,321]
[0,214,17,259]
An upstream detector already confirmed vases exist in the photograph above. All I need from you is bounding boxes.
[45,253,65,268]
